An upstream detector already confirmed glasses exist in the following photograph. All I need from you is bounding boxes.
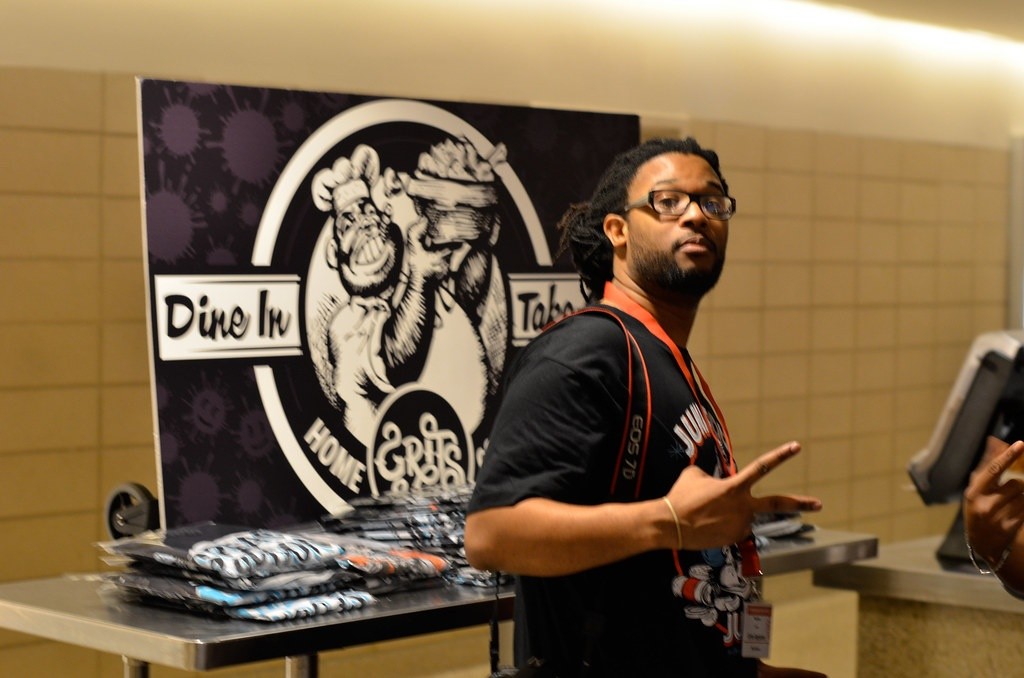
[613,189,736,222]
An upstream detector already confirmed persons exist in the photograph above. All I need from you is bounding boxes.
[962,345,1024,603]
[464,135,830,678]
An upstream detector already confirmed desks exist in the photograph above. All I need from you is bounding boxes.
[0,531,878,677]
[812,534,1024,677]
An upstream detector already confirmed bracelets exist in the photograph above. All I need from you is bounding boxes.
[662,495,682,549]
[965,536,1008,574]
